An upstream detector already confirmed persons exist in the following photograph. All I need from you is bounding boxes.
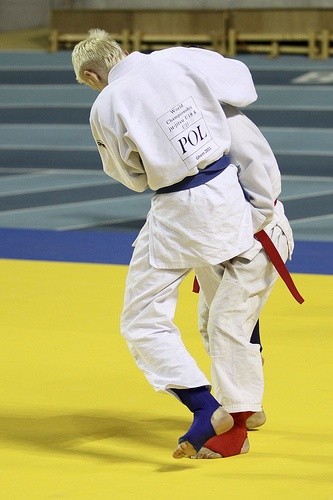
[71,28,257,459]
[192,104,304,459]
